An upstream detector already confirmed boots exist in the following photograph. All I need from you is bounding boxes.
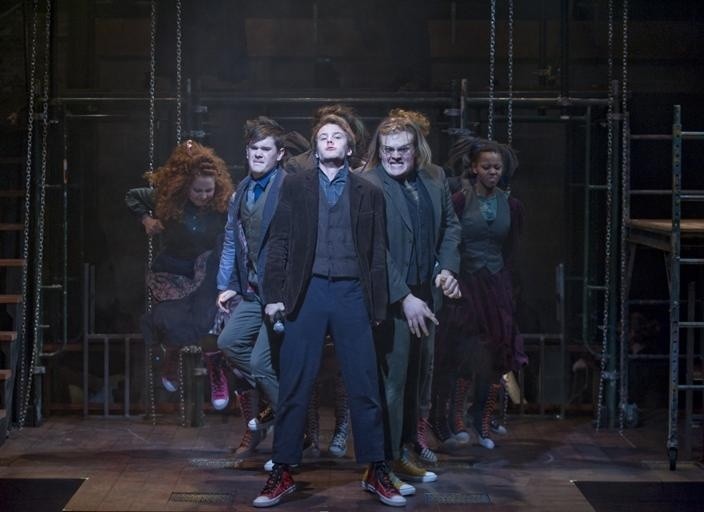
[162,362,180,393]
[412,414,439,464]
[233,389,267,459]
[472,383,502,450]
[449,376,471,444]
[327,372,351,459]
[204,350,229,411]
[433,389,457,446]
[302,375,321,457]
[256,394,312,452]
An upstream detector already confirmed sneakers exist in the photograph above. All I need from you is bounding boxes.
[361,464,416,497]
[263,457,301,475]
[252,464,298,508]
[488,413,509,437]
[367,461,406,507]
[247,401,281,431]
[385,456,438,483]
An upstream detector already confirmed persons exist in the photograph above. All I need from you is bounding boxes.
[217,117,288,457]
[253,116,408,507]
[282,129,309,172]
[288,106,362,459]
[447,145,530,450]
[392,108,433,137]
[126,140,233,410]
[359,118,463,483]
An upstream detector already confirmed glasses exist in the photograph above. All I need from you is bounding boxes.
[380,146,417,156]
